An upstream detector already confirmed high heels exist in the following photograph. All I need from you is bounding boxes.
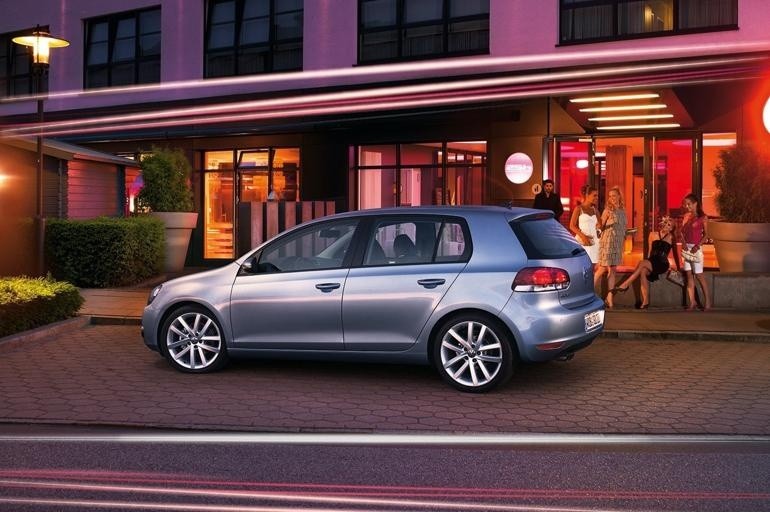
[640,304,649,310]
[616,286,629,294]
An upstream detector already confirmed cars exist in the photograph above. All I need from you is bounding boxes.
[140,203,607,395]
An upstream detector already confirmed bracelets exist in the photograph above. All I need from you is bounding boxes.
[575,229,581,235]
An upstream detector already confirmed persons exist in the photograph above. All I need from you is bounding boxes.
[678,193,712,313]
[593,185,628,311]
[570,184,602,266]
[534,179,564,222]
[615,216,679,311]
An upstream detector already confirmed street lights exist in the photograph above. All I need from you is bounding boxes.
[12,24,73,277]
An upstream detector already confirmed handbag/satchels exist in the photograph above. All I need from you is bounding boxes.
[576,233,595,246]
[681,247,704,263]
[667,268,685,287]
